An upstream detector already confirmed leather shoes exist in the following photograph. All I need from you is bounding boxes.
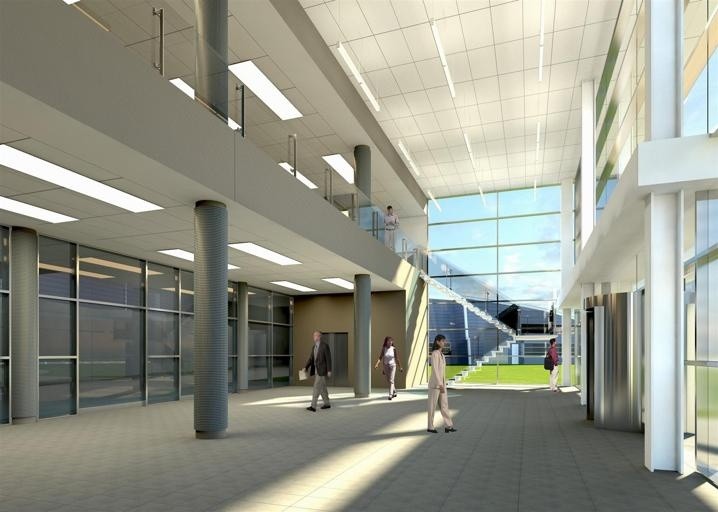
[307,406,316,412]
[321,405,330,409]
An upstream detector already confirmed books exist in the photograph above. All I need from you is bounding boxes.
[298,368,309,381]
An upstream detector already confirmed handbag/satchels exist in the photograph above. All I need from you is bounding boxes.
[544,356,553,370]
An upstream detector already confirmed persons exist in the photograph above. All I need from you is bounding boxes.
[383,205,399,249]
[426,335,457,433]
[374,337,403,399]
[547,338,559,391]
[302,330,332,411]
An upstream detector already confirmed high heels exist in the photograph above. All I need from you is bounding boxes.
[445,427,457,433]
[388,394,397,400]
[427,428,439,433]
